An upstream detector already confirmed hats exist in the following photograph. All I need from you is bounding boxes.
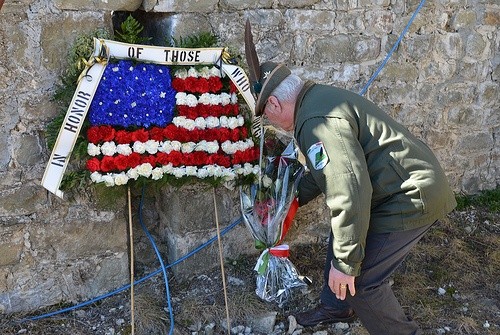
[255,61,291,117]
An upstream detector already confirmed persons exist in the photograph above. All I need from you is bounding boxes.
[249,61,458,335]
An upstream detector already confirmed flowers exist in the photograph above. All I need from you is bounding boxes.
[86,65,303,302]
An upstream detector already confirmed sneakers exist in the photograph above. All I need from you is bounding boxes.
[296,304,356,327]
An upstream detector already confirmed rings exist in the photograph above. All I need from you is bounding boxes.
[341,285,347,288]
